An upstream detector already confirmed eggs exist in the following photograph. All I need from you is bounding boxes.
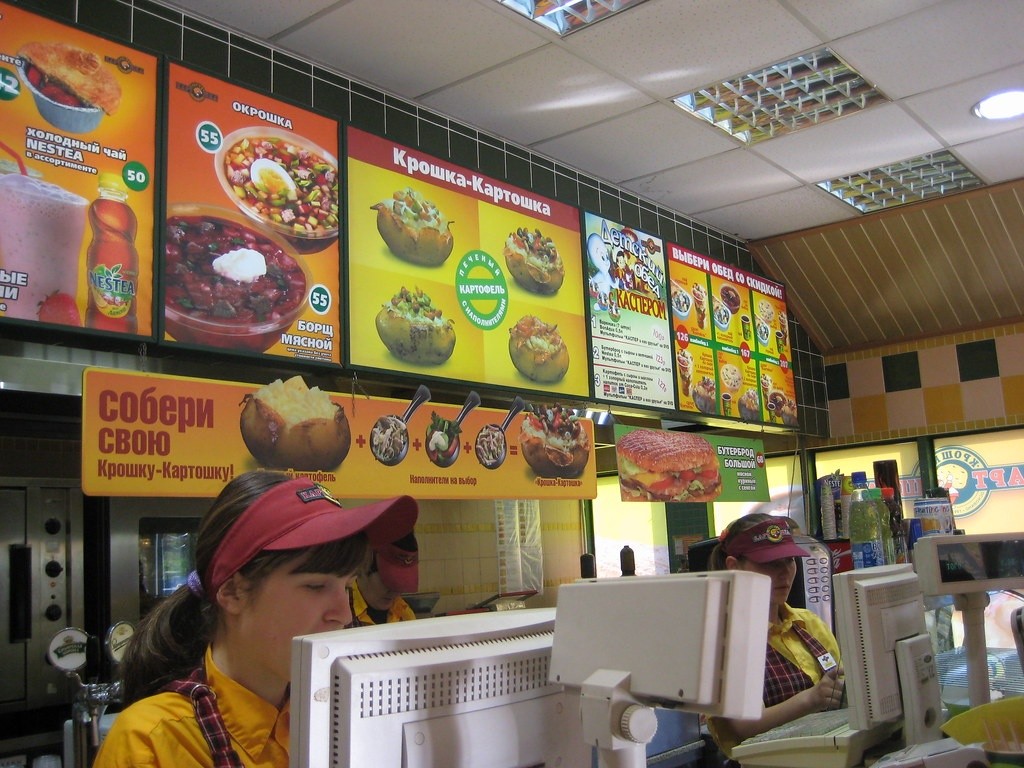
[251,158,297,199]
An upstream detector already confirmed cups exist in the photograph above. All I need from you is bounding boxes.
[690,289,707,329]
[775,331,784,355]
[763,382,774,409]
[768,402,777,424]
[741,316,752,341]
[819,475,855,541]
[677,356,694,396]
[722,392,733,417]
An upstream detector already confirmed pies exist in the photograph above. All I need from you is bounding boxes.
[20,41,122,117]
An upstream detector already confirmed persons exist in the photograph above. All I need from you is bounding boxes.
[703,512,847,761]
[89,472,420,768]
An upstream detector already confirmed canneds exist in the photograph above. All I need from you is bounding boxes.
[912,497,953,536]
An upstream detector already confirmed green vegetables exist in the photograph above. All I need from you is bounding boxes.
[430,410,462,436]
[173,220,290,324]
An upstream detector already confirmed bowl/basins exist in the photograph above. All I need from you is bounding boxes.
[766,390,787,417]
[754,316,770,346]
[17,52,108,132]
[710,295,731,331]
[213,124,343,254]
[670,288,694,320]
[165,201,315,353]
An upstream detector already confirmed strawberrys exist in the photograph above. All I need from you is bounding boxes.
[36,290,83,327]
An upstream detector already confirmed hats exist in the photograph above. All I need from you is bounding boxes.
[726,519,811,563]
[204,478,419,601]
[377,542,419,593]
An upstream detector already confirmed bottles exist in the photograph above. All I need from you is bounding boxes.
[848,485,909,572]
[85,170,139,334]
[0,171,90,326]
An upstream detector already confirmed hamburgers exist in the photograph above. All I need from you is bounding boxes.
[617,429,722,500]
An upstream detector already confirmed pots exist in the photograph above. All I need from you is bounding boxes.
[369,383,527,470]
